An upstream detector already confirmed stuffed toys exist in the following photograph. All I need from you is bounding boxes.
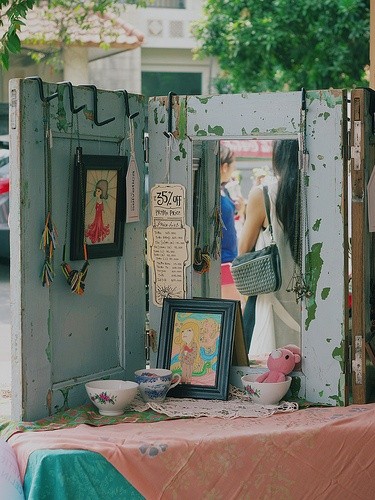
[280,344,301,371]
[255,349,299,384]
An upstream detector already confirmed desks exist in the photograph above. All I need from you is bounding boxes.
[0,399,375,500]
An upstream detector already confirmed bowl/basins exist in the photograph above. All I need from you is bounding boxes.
[241,374,292,405]
[85,380,139,416]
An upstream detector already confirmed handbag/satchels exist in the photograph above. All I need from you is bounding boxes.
[220,264,234,286]
[230,184,282,296]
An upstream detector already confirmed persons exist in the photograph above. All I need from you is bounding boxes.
[238,139,302,367]
[219,142,239,312]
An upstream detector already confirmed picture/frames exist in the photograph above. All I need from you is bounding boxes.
[69,153,128,260]
[156,298,238,400]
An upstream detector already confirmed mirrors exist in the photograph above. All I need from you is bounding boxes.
[188,134,303,372]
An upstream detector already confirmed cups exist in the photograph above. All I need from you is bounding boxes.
[134,369,181,404]
